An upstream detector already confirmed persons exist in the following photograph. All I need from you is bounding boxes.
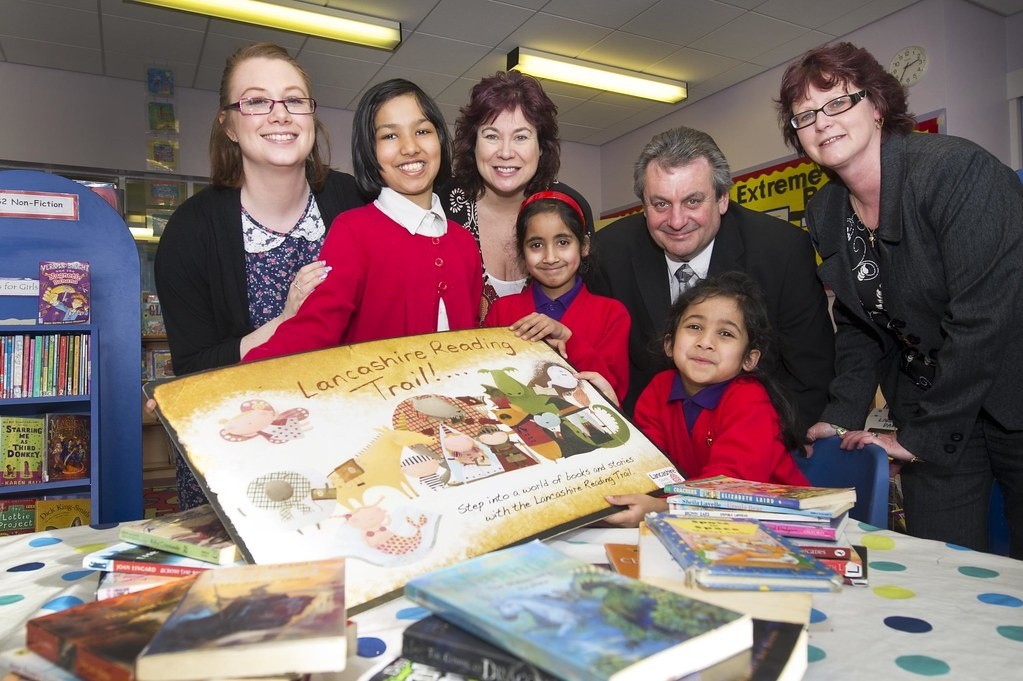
[156,46,1022,558]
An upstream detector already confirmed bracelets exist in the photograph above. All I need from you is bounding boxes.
[831,424,848,435]
[911,456,919,463]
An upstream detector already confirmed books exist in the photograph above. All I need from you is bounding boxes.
[0,260,868,681]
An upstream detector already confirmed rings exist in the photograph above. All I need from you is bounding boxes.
[293,281,301,290]
[871,431,877,437]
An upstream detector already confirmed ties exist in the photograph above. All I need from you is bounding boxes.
[674,264,695,294]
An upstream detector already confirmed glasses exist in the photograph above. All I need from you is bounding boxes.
[790,89,872,129]
[223,97,317,115]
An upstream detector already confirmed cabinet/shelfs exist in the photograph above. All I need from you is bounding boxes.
[1,324,99,538]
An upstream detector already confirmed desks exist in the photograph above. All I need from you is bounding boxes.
[0,518,1023,681]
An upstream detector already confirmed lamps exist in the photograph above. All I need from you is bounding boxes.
[507,47,688,105]
[124,0,402,51]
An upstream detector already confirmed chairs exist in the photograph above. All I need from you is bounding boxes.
[791,435,889,528]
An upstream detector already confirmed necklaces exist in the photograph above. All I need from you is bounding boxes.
[853,194,878,248]
